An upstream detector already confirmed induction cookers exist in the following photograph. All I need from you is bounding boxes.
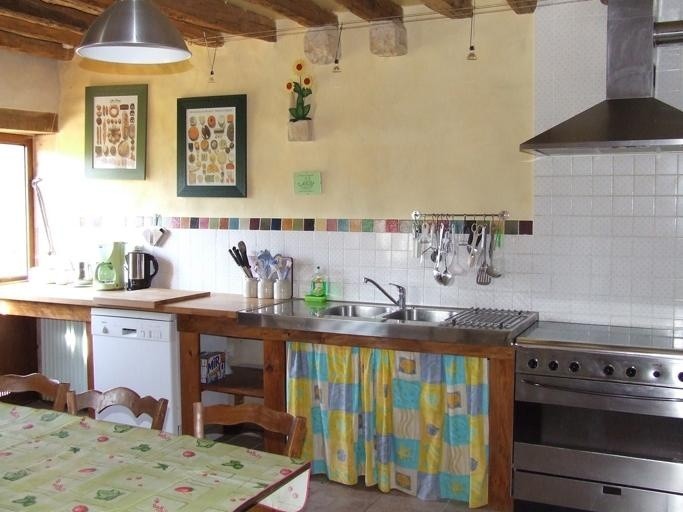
[510,315,682,385]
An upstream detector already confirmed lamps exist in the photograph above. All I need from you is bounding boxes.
[76,2,191,64]
[332,24,343,72]
[209,41,217,82]
[467,12,478,59]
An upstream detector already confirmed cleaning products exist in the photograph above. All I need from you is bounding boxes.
[310,267,327,296]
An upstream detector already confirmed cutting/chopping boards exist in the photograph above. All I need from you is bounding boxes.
[91,288,210,309]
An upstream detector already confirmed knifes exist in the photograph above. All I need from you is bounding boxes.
[226,241,253,278]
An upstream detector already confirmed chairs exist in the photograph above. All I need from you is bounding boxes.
[67,387,168,430]
[0,373,69,412]
[194,402,305,511]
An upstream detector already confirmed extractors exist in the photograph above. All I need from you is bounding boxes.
[519,0,681,157]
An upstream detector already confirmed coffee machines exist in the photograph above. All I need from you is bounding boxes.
[91,241,126,291]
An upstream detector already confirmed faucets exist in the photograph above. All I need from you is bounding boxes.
[364,278,405,309]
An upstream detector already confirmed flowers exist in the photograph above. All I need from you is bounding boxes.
[284,59,316,120]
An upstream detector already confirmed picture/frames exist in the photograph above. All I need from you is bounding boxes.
[84,84,148,180]
[176,93,248,198]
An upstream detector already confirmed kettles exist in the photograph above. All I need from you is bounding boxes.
[124,250,158,290]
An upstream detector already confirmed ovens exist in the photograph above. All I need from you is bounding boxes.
[509,370,682,512]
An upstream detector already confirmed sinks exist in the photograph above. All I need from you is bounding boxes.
[317,303,387,321]
[382,308,459,323]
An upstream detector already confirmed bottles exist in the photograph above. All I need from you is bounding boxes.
[243,298,258,315]
[256,277,272,300]
[274,300,293,319]
[243,277,256,299]
[258,297,273,316]
[273,278,292,298]
